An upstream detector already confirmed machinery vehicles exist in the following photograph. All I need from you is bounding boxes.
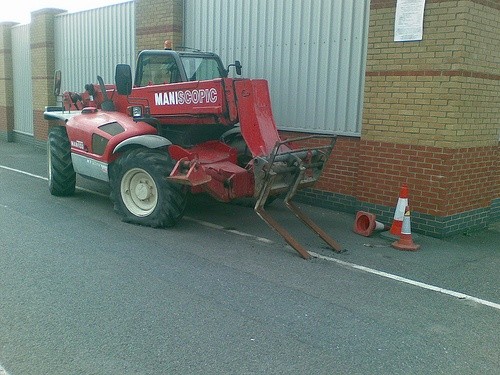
[43,39,341,259]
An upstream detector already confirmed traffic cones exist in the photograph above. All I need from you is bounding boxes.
[380,183,409,240]
[353,211,390,237]
[391,206,419,251]
